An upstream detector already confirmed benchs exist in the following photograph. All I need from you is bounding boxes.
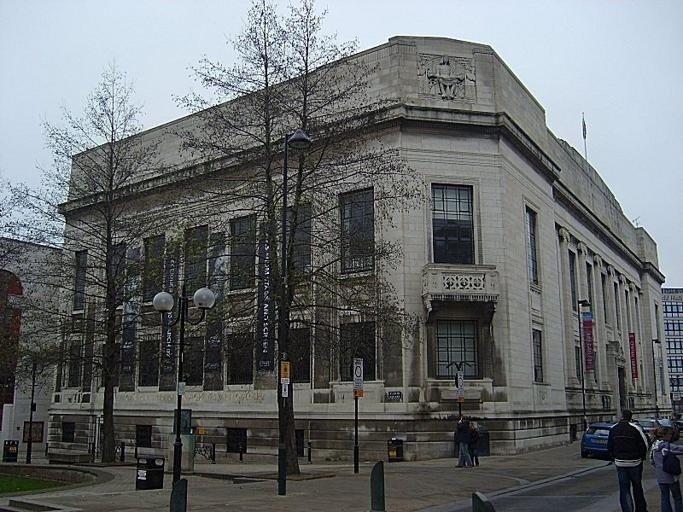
[45,451,91,466]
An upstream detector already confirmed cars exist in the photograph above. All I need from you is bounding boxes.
[580,412,682,461]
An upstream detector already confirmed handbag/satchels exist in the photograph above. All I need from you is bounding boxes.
[663,452,681,475]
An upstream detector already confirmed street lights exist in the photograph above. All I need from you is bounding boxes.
[651,339,661,417]
[153,281,217,481]
[576,297,591,428]
[23,343,40,463]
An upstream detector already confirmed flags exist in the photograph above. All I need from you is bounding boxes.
[581,118,588,139]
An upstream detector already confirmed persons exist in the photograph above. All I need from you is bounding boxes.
[606,408,649,511]
[648,425,682,511]
[454,414,473,468]
[468,422,480,467]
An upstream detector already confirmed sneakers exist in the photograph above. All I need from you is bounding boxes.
[455,463,480,468]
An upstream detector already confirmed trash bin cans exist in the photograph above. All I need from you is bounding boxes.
[136,456,165,490]
[387,438,404,463]
[3,440,19,462]
